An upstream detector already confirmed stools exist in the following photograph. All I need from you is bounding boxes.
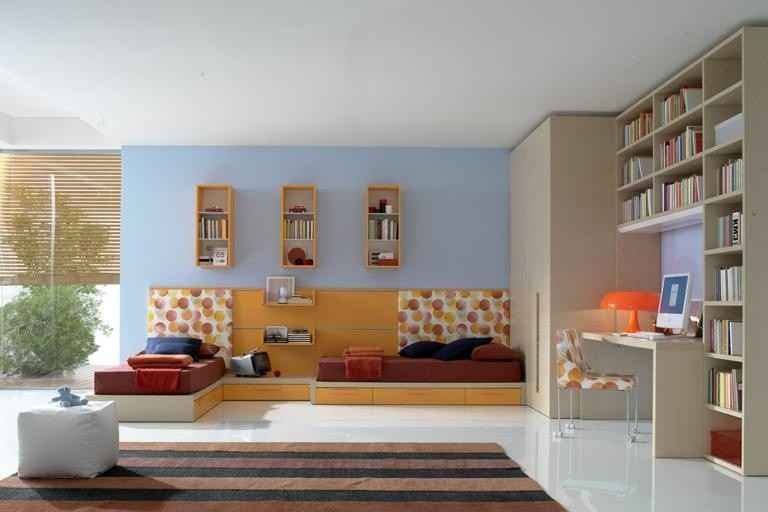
[16,399,121,479]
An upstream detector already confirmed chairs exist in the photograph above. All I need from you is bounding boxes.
[553,328,640,446]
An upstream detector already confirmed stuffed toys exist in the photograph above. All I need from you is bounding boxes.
[50,384,89,407]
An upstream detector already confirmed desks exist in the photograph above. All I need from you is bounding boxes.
[582,330,704,459]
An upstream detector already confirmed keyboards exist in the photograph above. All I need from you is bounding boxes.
[629,331,664,340]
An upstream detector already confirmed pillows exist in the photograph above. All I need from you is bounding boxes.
[397,337,521,361]
[127,336,221,362]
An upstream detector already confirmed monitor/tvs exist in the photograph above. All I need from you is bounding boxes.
[655,271,696,339]
[231,352,271,376]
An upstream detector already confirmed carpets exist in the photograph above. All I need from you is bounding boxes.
[0,442,569,512]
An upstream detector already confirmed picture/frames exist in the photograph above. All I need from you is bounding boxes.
[265,276,294,304]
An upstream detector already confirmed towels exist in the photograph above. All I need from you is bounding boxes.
[127,354,193,391]
[342,345,384,380]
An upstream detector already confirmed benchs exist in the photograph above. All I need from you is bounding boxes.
[85,356,225,422]
[310,353,526,405]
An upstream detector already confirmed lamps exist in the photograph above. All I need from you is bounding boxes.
[599,290,661,332]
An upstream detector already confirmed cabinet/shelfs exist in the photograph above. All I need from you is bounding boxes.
[614,25,768,476]
[364,185,401,269]
[261,287,316,346]
[280,184,317,268]
[194,182,234,269]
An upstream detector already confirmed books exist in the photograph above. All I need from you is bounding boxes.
[212,247,228,265]
[267,332,313,343]
[283,217,313,240]
[199,215,228,239]
[368,217,397,266]
